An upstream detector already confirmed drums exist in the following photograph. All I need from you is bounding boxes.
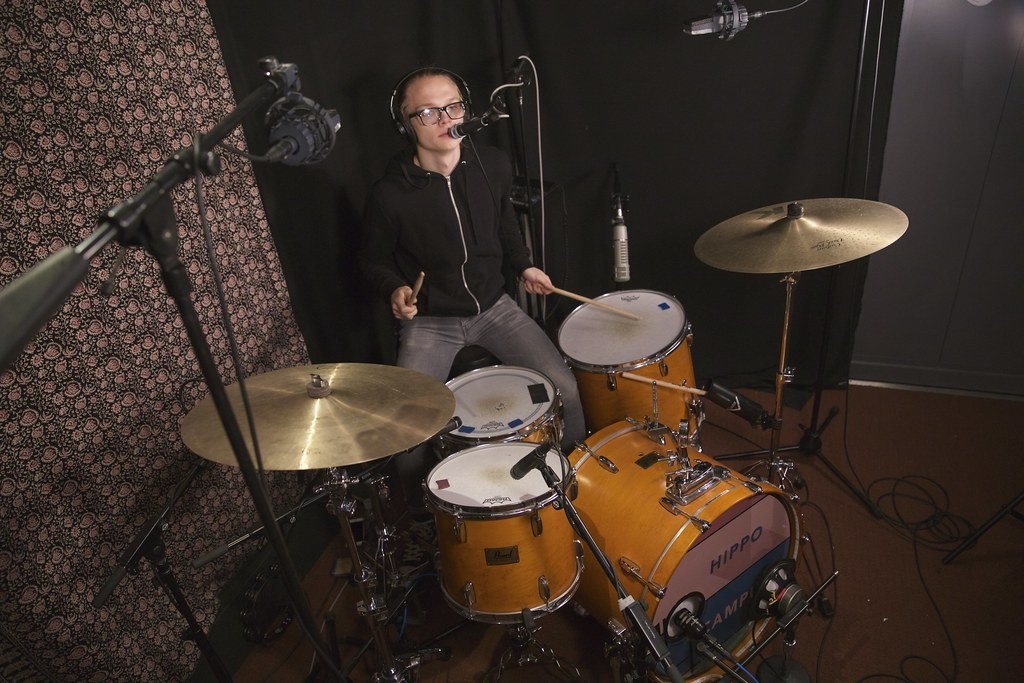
[557,288,707,448]
[423,440,585,625]
[431,364,566,462]
[567,413,812,682]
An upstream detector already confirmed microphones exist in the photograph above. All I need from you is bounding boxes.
[447,112,499,138]
[610,181,631,282]
[682,0,768,42]
[264,91,342,165]
[510,439,554,479]
[392,416,462,457]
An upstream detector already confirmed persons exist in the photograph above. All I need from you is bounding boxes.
[363,67,586,592]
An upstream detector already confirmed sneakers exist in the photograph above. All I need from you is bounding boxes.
[399,520,437,578]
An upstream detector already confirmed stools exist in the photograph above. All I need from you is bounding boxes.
[451,346,496,372]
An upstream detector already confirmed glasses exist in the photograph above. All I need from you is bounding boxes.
[409,101,467,126]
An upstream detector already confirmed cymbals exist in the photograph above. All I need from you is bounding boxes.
[692,195,912,276]
[178,361,458,473]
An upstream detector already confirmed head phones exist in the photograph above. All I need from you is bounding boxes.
[389,65,475,145]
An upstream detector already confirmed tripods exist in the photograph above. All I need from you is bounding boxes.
[192,446,474,683]
[712,0,886,519]
